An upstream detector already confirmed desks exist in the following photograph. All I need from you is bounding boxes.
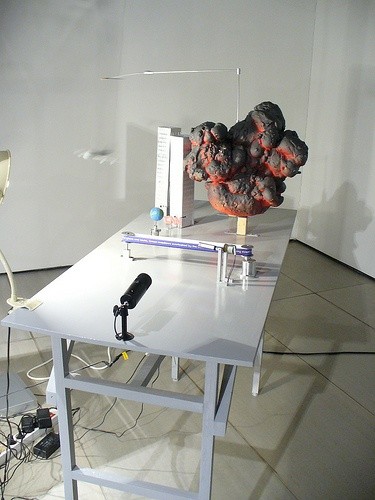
[0,199,296,500]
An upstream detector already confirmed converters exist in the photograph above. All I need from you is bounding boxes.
[21,416,33,433]
[37,409,52,429]
[31,432,59,459]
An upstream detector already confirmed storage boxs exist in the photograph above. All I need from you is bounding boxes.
[168,133,195,229]
[155,126,180,222]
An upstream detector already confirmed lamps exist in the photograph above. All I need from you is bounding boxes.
[114,271,152,339]
[98,67,240,123]
[0,149,42,312]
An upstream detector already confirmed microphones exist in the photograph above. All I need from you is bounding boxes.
[120,273,152,310]
